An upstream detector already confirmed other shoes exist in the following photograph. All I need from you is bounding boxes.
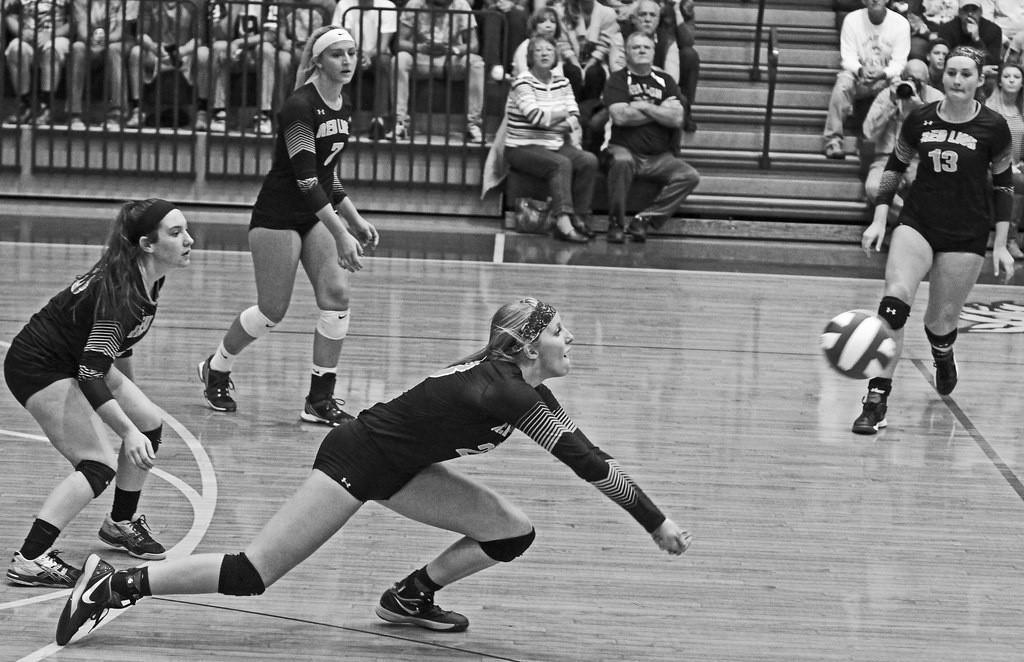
[607,224,624,243]
[1008,240,1024,259]
[251,114,274,134]
[626,217,648,243]
[467,125,487,144]
[101,111,123,125]
[554,222,588,243]
[9,102,49,125]
[573,218,595,237]
[196,110,207,131]
[685,117,696,132]
[826,138,847,159]
[210,110,225,132]
[385,122,411,140]
[65,115,84,127]
[127,109,146,128]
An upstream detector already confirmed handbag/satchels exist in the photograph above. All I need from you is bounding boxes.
[515,197,555,236]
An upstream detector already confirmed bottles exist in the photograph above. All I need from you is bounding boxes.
[164,30,184,68]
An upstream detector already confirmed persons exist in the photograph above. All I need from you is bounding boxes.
[3,197,196,589]
[1,0,1024,433]
[54,297,691,646]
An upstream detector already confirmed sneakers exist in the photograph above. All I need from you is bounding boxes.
[98,514,167,561]
[852,385,892,434]
[55,553,125,646]
[198,354,237,412]
[932,348,957,395]
[300,394,356,426]
[4,547,82,588]
[375,575,469,632]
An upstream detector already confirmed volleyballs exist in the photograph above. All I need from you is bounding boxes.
[820,304,894,384]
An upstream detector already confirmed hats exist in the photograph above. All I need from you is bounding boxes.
[958,0,981,10]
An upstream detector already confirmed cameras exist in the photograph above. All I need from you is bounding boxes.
[896,76,922,100]
